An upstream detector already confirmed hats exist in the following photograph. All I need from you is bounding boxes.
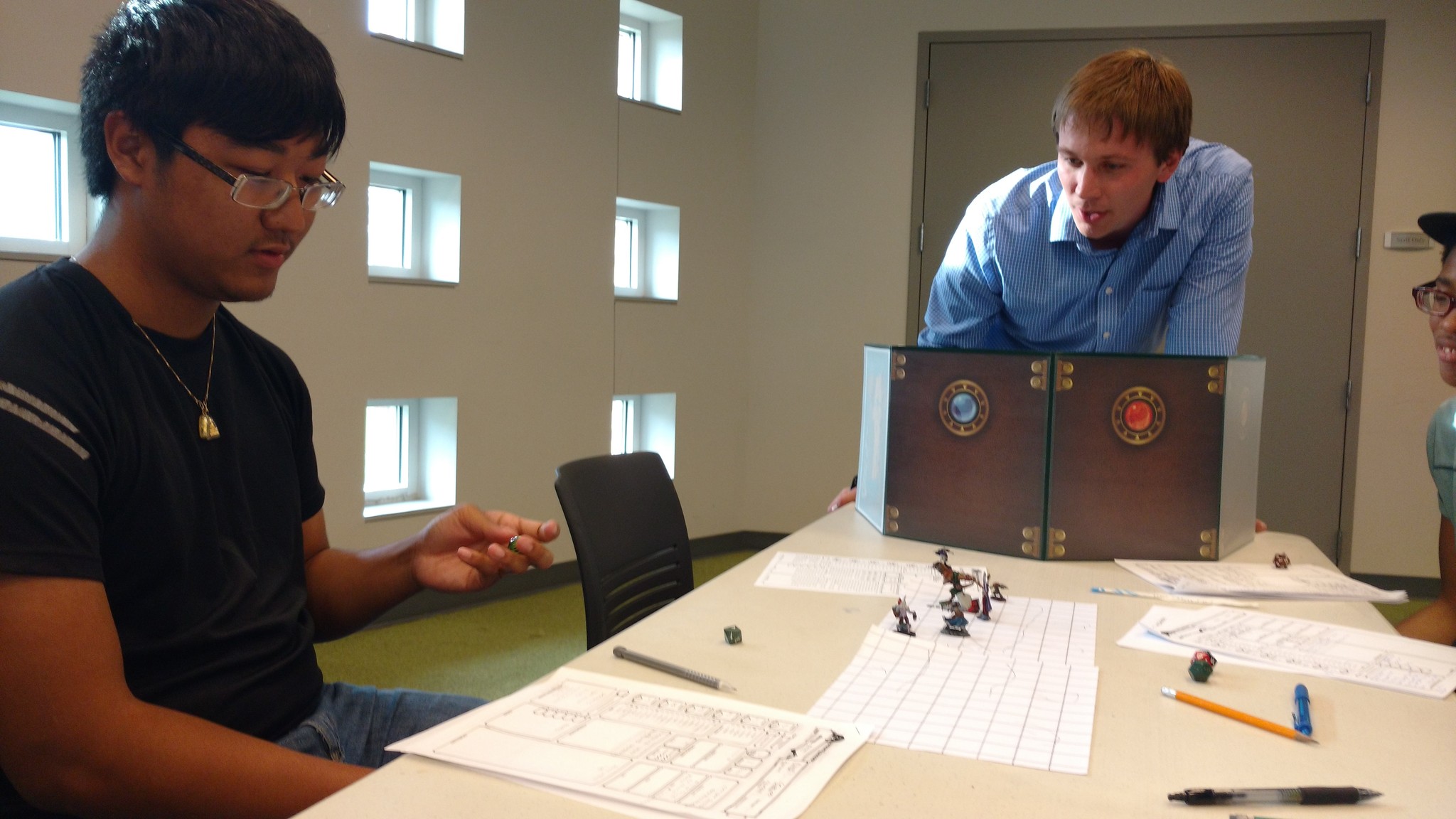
[1418,212,1456,246]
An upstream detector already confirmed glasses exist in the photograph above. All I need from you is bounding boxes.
[151,125,344,210]
[1412,280,1456,317]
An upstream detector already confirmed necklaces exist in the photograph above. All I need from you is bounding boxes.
[71,255,220,439]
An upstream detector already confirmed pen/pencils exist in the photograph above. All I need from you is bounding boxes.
[1162,685,1319,744]
[1292,684,1312,737]
[613,646,738,692]
[1168,787,1382,806]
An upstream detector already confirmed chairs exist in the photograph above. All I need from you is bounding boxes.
[555,450,697,653]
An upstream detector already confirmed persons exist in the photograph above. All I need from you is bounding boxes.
[1392,212,1456,649]
[829,50,1254,513]
[0,0,561,819]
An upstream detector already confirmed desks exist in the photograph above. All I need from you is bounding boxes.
[287,503,1456,819]
